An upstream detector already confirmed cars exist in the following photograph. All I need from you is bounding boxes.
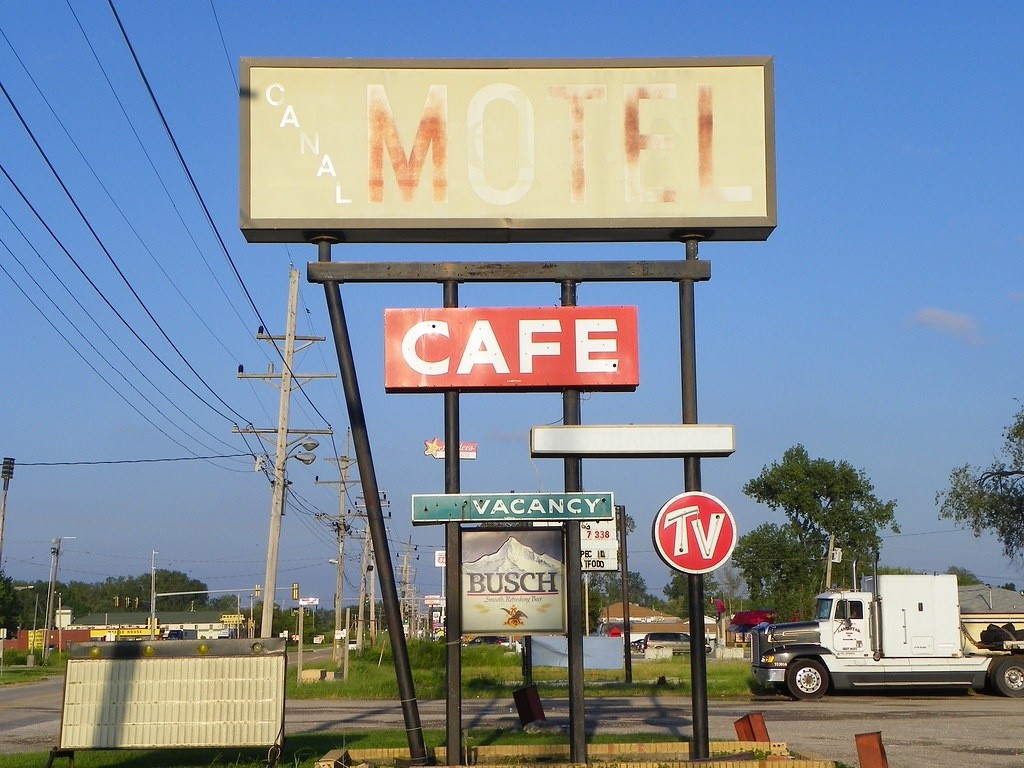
[644,632,713,655]
[630,639,644,652]
[466,635,502,646]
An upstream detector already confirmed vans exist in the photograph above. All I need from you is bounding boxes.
[349,639,358,650]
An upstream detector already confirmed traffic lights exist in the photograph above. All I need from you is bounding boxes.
[404,609,409,624]
[364,594,369,604]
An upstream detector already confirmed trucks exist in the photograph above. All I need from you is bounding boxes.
[747,550,1023,701]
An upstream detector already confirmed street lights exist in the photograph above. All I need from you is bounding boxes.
[151,551,161,641]
[45,536,78,658]
